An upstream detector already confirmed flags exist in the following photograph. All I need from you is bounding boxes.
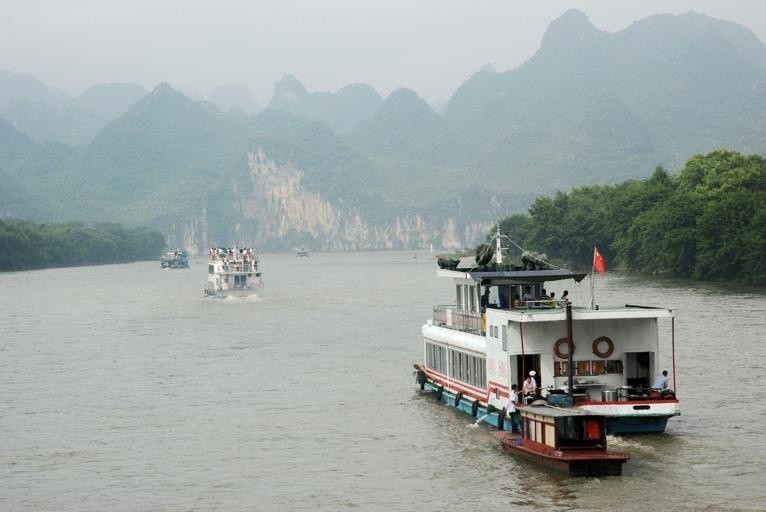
[593,250,605,278]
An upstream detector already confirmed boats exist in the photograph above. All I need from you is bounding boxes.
[413,220,680,435]
[489,392,631,476]
[160,246,188,268]
[206,244,264,298]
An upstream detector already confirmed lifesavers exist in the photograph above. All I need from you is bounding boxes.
[553,338,575,358]
[592,336,613,358]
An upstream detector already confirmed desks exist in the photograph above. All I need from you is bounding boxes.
[573,384,606,403]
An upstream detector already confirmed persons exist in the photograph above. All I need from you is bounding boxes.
[209,247,254,257]
[529,370,546,395]
[523,376,539,403]
[508,383,525,433]
[651,370,669,391]
[482,285,569,307]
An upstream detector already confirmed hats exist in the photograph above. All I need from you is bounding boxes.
[529,370,536,375]
